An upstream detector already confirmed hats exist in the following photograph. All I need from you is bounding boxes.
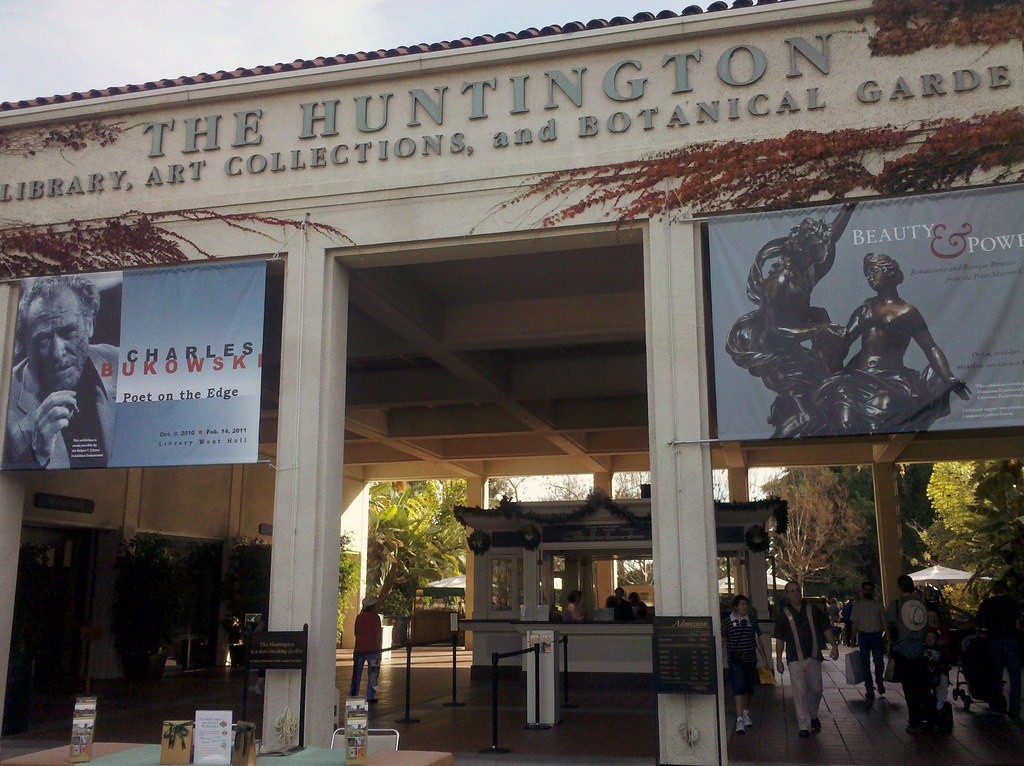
[362,596,378,609]
[901,600,927,631]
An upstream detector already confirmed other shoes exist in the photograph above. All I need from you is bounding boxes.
[907,725,922,733]
[367,699,378,704]
[866,688,875,699]
[877,680,886,695]
[811,717,821,730]
[799,730,810,738]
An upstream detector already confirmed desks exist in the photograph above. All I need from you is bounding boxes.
[0,742,455,766]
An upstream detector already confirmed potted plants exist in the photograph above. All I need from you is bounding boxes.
[106,532,187,681]
[215,534,269,666]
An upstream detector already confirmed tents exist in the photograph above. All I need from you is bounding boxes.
[405,574,507,644]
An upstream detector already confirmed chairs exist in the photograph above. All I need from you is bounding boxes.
[329,728,400,750]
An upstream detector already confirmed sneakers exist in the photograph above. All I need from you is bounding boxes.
[743,714,752,727]
[736,722,745,736]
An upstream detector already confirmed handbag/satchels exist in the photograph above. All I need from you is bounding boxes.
[846,651,865,686]
[885,658,900,683]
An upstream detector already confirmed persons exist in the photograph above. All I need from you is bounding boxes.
[825,597,858,648]
[606,587,648,621]
[773,581,838,738]
[948,614,976,663]
[885,574,952,735]
[247,601,270,695]
[849,582,889,699]
[720,594,763,733]
[566,590,584,620]
[745,201,973,439]
[349,597,382,703]
[2,276,120,470]
[976,580,1024,719]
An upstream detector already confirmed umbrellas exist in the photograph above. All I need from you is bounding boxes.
[906,565,992,581]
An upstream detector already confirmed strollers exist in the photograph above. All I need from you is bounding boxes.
[953,632,1008,711]
